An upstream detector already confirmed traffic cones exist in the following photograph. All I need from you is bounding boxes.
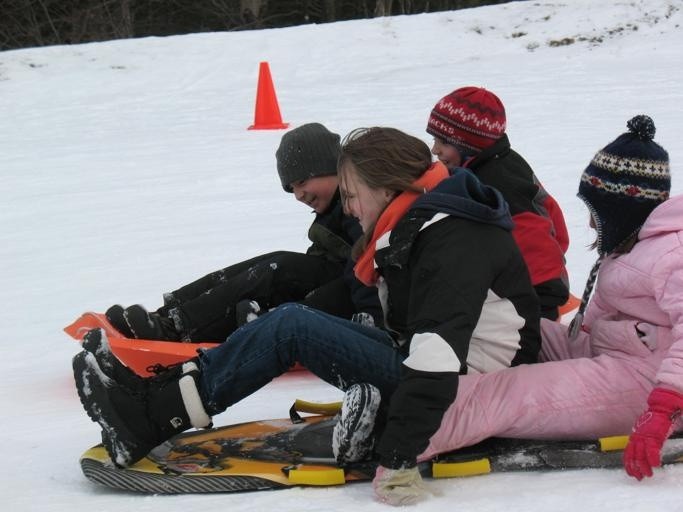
[248,61,288,130]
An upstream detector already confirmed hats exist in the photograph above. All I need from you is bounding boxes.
[567,115,671,338]
[275,122,340,193]
[426,87,506,154]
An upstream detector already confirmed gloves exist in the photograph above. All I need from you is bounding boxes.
[372,466,444,506]
[623,387,683,481]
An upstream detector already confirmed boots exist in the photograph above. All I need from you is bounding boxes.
[332,383,382,464]
[72,328,213,468]
[104,293,186,339]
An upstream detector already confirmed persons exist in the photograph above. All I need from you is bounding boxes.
[105,122,383,339]
[234,88,569,329]
[72,127,541,506]
[331,115,683,481]
